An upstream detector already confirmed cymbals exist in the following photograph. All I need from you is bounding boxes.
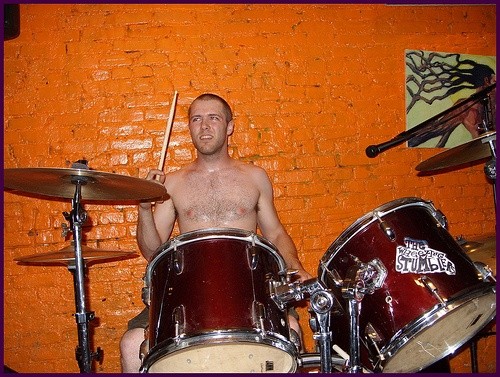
[11,241,137,266]
[1,167,167,207]
[414,129,498,173]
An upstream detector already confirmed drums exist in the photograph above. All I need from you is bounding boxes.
[139,227,299,373]
[315,196,498,374]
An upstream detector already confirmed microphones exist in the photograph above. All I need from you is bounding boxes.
[365,132,416,158]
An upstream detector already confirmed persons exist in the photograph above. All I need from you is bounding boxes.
[441,64,496,138]
[120,94,312,373]
